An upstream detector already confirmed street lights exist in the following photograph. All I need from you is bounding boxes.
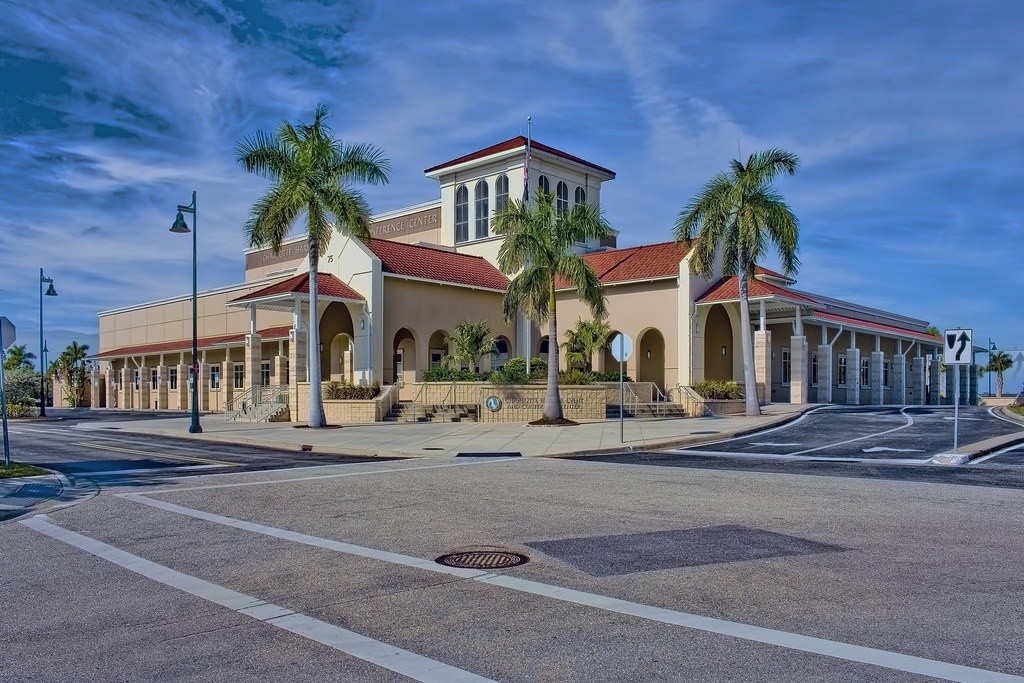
[988,336,998,398]
[43,338,50,407]
[169,190,206,434]
[37,267,59,414]
